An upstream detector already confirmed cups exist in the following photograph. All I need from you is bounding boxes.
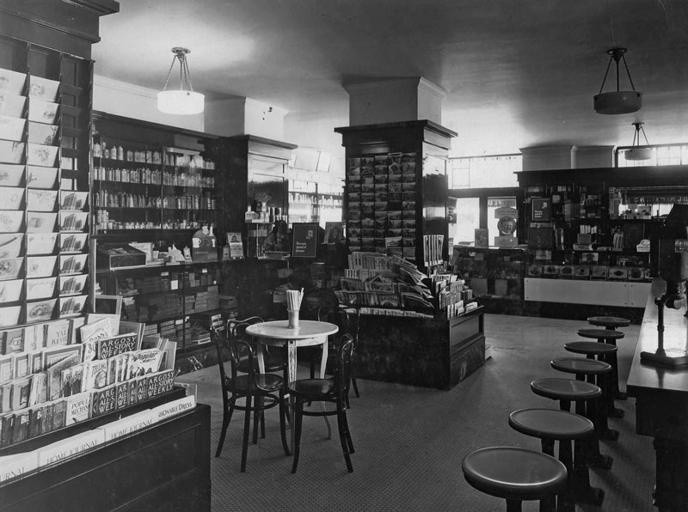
[287,309,299,329]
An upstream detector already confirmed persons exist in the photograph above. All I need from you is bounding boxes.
[261,220,291,251]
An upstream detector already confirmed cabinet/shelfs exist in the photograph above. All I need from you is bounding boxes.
[521,186,688,282]
[285,168,345,229]
[452,247,525,315]
[96,258,346,376]
[90,117,219,239]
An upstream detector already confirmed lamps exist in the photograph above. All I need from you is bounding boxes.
[625,122,651,160]
[151,47,208,116]
[593,47,641,115]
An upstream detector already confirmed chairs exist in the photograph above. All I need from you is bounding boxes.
[307,305,359,406]
[287,339,357,475]
[215,340,290,473]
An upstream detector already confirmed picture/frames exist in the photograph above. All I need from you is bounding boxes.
[225,231,245,260]
[291,222,319,259]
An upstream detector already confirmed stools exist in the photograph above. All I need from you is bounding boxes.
[460,316,631,512]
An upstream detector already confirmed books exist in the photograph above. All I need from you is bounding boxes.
[0,70,238,451]
[523,194,657,282]
[333,150,479,322]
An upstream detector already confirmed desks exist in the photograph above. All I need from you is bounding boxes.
[245,319,337,449]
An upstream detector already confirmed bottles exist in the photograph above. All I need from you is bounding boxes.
[110,144,124,160]
